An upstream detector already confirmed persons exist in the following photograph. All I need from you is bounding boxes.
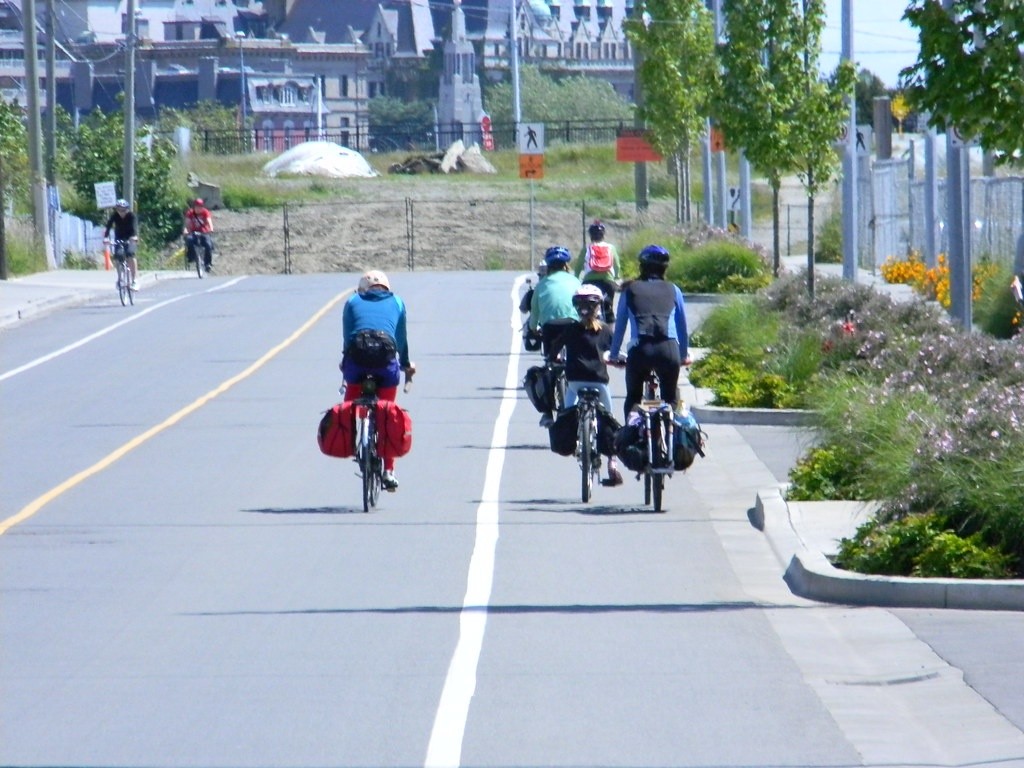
[548,284,627,485]
[182,198,213,272]
[608,245,692,425]
[104,199,138,290]
[339,270,416,487]
[519,260,547,314]
[573,224,621,333]
[523,247,602,427]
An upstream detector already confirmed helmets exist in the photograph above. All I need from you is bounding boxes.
[116,199,129,209]
[358,270,390,291]
[545,245,570,266]
[639,245,669,266]
[589,224,606,233]
[194,199,203,206]
[572,284,604,304]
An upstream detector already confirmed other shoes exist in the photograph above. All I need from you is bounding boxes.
[608,461,623,484]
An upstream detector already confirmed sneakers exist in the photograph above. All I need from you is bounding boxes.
[540,414,555,429]
[382,470,398,487]
[131,281,138,291]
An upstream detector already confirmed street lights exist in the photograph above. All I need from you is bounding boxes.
[235,31,246,153]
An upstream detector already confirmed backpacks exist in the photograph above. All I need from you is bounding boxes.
[316,400,356,458]
[615,410,649,481]
[376,399,411,460]
[523,365,557,412]
[595,399,623,458]
[549,403,579,456]
[590,241,613,272]
[659,405,707,471]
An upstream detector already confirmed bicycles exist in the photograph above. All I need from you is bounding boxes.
[342,363,412,513]
[523,281,688,514]
[108,240,138,307]
[189,231,205,278]
